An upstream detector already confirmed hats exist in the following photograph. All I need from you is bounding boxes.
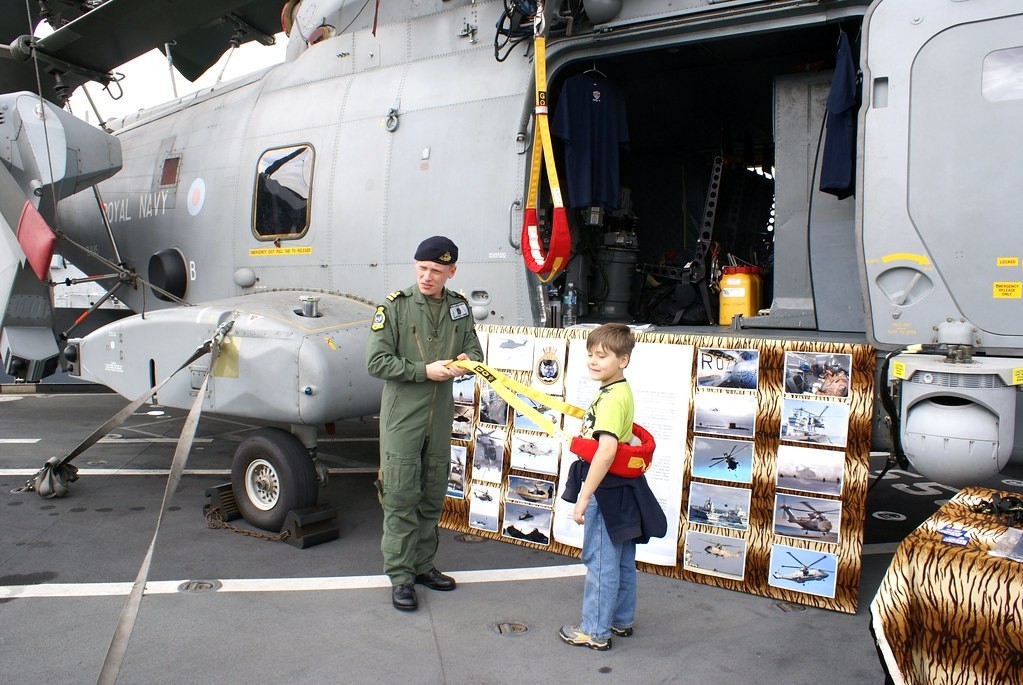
[415,236,458,265]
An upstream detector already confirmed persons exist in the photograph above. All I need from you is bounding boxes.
[366,236,484,611]
[559,323,638,651]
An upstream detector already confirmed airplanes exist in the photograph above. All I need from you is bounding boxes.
[0,1,1023,533]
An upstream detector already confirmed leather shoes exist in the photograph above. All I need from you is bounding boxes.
[414,567,456,591]
[392,584,417,609]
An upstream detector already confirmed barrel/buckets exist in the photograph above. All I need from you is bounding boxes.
[594,247,640,317]
[718,265,762,326]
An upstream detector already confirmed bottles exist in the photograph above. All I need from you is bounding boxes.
[562,283,577,329]
[537,282,552,327]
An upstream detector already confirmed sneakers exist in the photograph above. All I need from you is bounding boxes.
[559,625,612,651]
[609,624,633,637]
